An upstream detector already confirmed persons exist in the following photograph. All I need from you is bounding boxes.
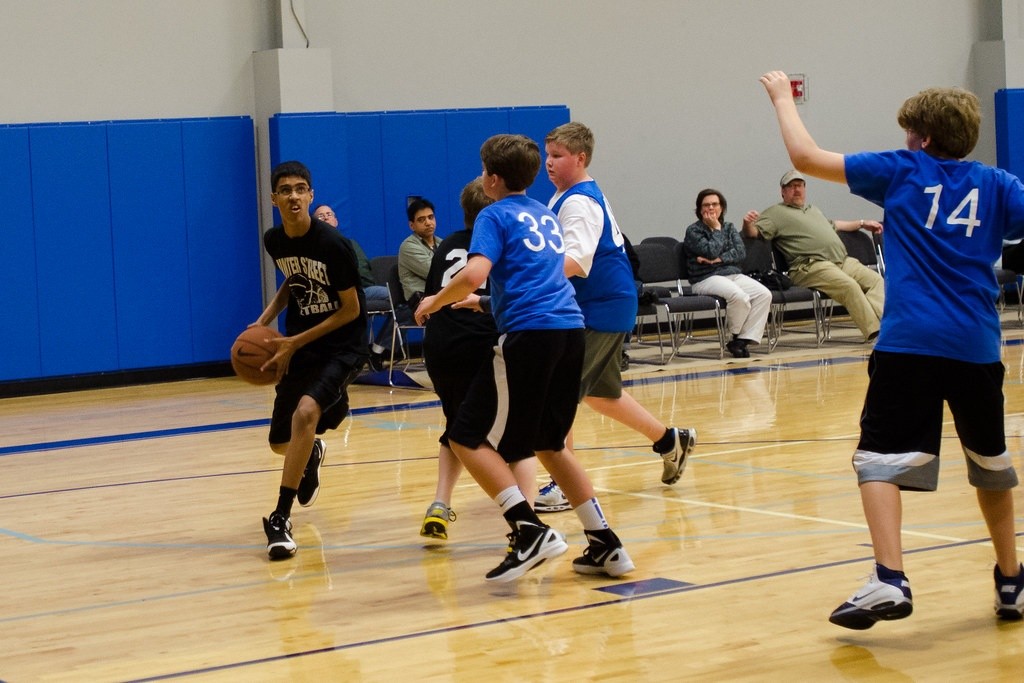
[247,160,360,559]
[684,189,772,358]
[415,121,695,583]
[311,199,443,372]
[619,231,644,371]
[759,71,1024,629]
[743,170,884,348]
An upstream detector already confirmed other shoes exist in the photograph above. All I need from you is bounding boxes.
[621,355,630,371]
[726,341,750,358]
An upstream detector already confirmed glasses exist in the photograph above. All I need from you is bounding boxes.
[317,214,334,220]
[274,186,311,195]
[702,202,720,207]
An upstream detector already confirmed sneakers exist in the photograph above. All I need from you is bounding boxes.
[533,475,574,512]
[293,525,334,595]
[367,343,384,374]
[993,561,1024,620]
[420,502,452,540]
[829,646,914,683]
[572,546,635,577]
[297,438,327,507]
[270,561,297,582]
[661,427,697,485]
[262,511,298,559]
[485,525,568,583]
[829,564,913,630]
[422,544,448,591]
[657,489,701,555]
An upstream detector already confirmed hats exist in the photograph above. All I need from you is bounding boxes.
[780,170,806,187]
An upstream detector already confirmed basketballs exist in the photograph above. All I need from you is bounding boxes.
[231,327,287,385]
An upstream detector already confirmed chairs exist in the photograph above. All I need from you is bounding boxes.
[629,221,885,365]
[993,254,1024,327]
[367,255,427,386]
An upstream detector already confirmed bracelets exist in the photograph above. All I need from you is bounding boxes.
[860,219,864,228]
[711,260,713,264]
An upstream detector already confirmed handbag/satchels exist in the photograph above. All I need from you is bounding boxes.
[747,268,792,291]
